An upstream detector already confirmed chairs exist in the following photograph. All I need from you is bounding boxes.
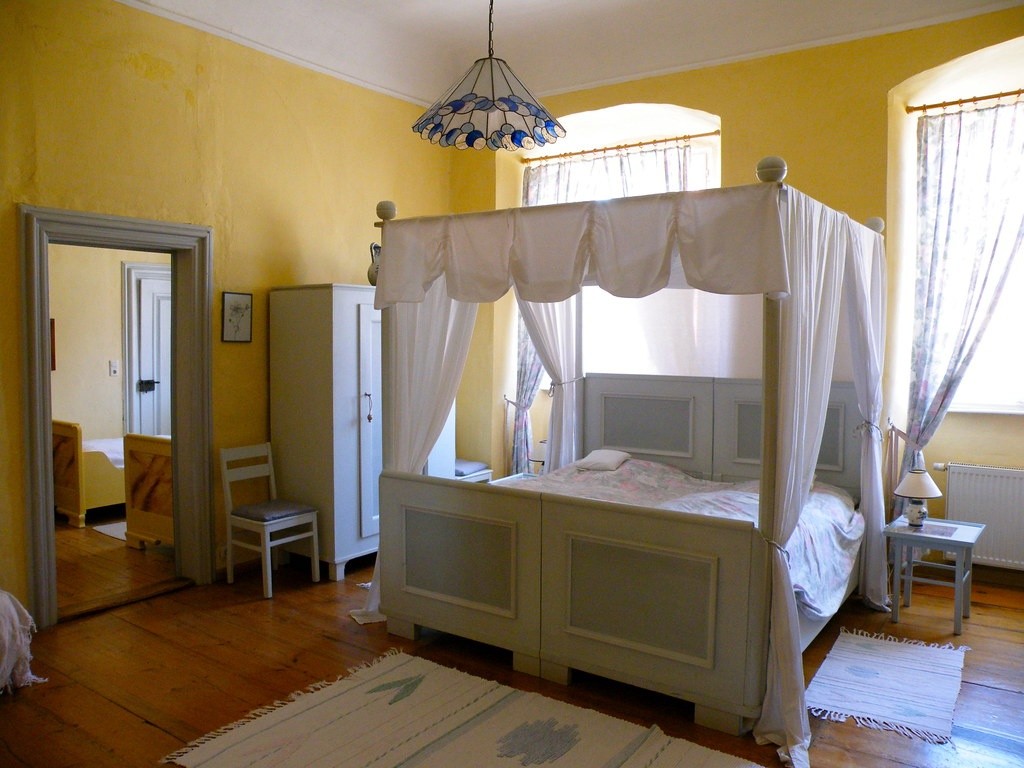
[219,443,321,601]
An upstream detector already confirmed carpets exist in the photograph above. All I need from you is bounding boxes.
[802,626,973,748]
[157,645,769,768]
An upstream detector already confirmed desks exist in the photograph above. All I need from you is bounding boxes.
[882,512,986,637]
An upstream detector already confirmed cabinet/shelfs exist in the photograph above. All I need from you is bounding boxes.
[266,282,457,583]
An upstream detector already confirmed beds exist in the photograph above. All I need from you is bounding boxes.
[52,420,174,551]
[371,154,891,768]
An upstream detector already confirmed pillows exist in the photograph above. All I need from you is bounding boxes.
[574,447,632,472]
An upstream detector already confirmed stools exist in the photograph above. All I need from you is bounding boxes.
[454,457,494,483]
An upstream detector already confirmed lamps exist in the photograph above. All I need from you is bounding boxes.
[410,0,567,151]
[894,468,945,529]
[527,440,548,477]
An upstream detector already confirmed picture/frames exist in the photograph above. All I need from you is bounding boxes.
[221,291,254,344]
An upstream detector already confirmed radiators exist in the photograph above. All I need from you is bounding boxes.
[942,463,1024,571]
